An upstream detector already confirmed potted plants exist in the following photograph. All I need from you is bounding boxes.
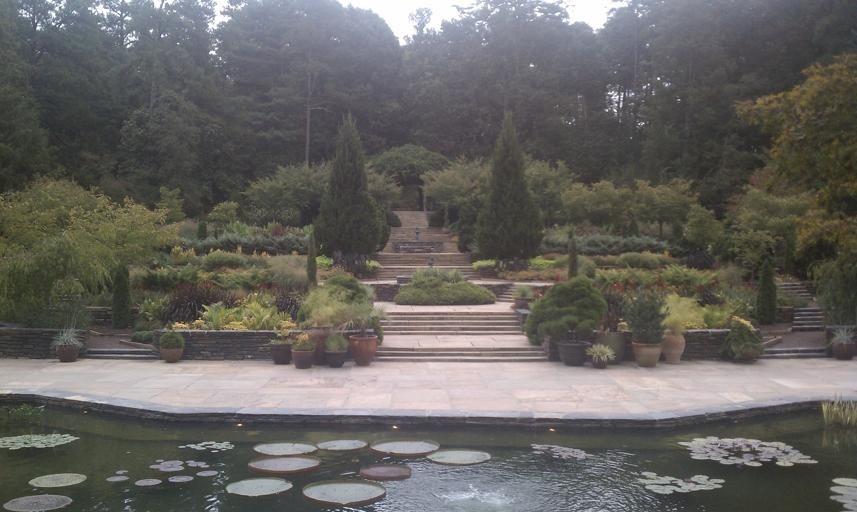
[50,308,86,363]
[49,270,83,330]
[813,257,857,360]
[521,267,700,370]
[269,273,389,371]
[136,294,199,364]
